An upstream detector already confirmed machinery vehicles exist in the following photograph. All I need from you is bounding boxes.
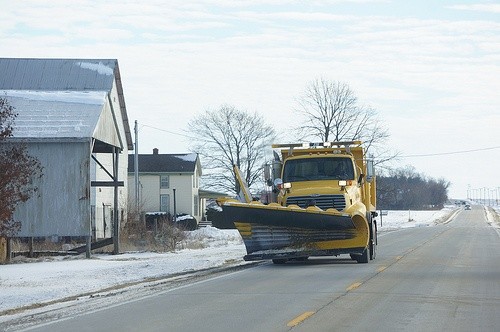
[221,140,379,263]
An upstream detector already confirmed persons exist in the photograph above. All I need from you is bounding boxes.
[335,163,348,176]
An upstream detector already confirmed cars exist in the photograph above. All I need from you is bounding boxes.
[465,205,471,210]
